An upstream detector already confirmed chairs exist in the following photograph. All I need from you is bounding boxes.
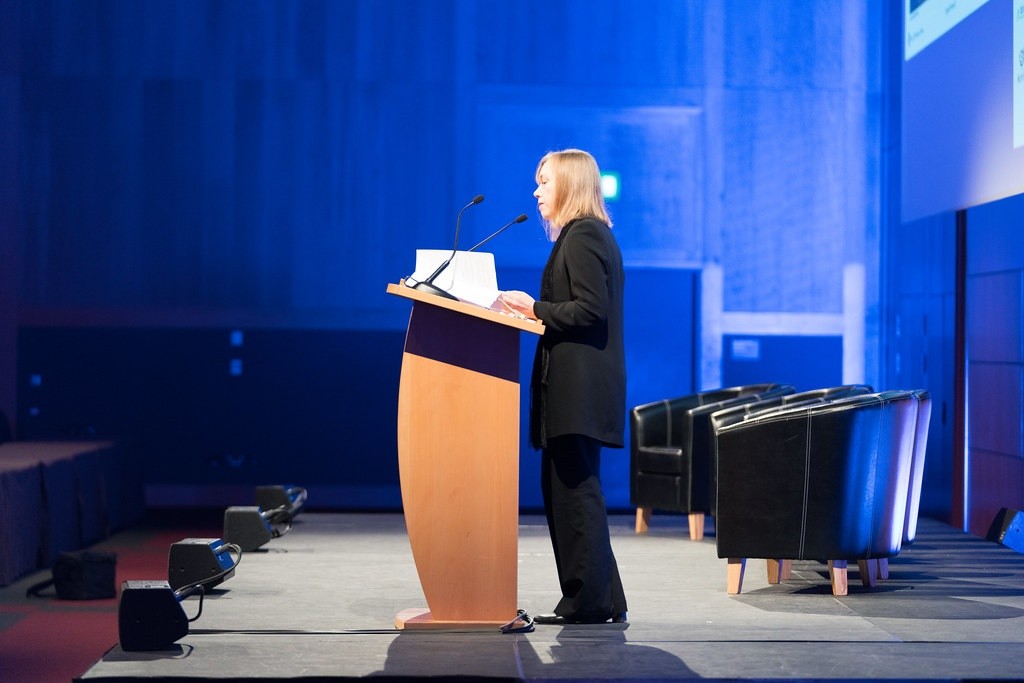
[709,384,932,579]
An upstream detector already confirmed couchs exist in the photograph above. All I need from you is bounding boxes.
[631,383,795,541]
[715,389,920,596]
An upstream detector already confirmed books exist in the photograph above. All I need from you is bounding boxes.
[406,249,498,308]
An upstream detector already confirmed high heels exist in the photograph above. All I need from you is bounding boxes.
[533,610,627,625]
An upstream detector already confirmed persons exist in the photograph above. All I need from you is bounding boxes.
[498,150,628,624]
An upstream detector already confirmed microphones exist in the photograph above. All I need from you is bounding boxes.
[466,213,529,252]
[426,194,485,284]
